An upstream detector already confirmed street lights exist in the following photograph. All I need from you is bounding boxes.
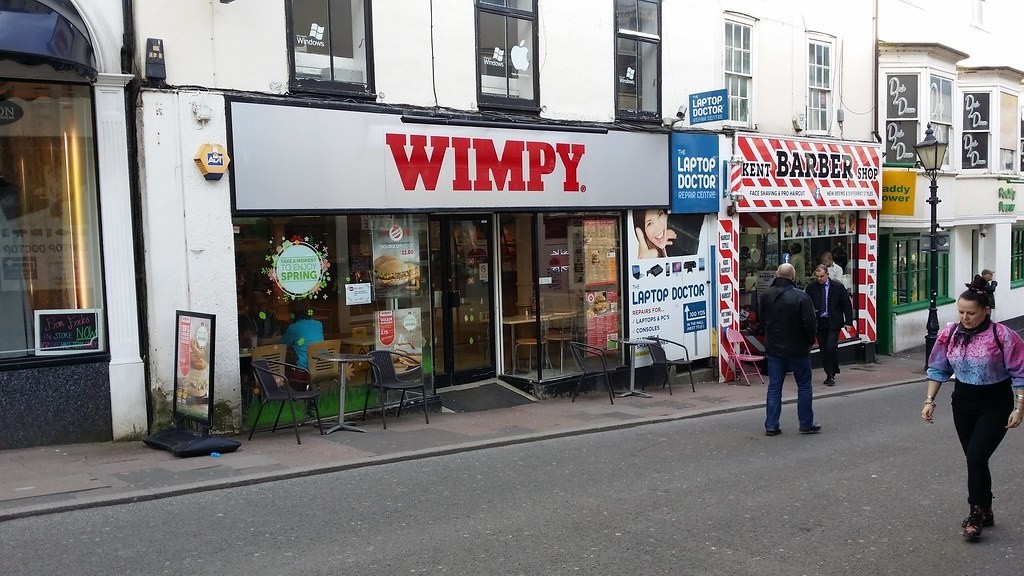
[913,122,949,371]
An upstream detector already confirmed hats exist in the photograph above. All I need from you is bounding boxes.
[982,269,995,275]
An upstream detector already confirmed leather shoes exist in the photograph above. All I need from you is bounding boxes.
[823,376,829,384]
[827,375,836,386]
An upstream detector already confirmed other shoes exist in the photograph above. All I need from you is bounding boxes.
[799,423,821,433]
[766,429,782,435]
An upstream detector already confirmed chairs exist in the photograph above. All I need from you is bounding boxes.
[306,338,341,405]
[364,350,429,429]
[545,313,582,373]
[723,330,765,386]
[512,317,549,375]
[251,359,323,444]
[570,341,617,406]
[252,344,287,388]
[645,337,696,396]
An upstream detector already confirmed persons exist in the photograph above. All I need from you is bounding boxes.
[848,213,855,231]
[806,264,853,386]
[783,216,792,238]
[795,214,804,237]
[973,269,997,316]
[828,215,836,234]
[238,292,280,377]
[821,252,843,282]
[922,274,1024,538]
[807,215,815,236]
[280,296,324,382]
[632,208,677,259]
[818,215,825,235]
[839,212,846,234]
[790,243,805,276]
[757,263,821,435]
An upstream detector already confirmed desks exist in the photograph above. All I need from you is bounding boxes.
[480,311,582,367]
[341,335,376,377]
[314,354,366,435]
[611,336,657,398]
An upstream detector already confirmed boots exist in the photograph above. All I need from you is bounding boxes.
[962,507,994,529]
[963,506,984,537]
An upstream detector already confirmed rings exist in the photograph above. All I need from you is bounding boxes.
[922,416,924,419]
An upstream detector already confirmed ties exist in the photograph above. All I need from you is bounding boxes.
[821,285,826,314]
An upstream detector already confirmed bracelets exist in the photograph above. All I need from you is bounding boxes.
[926,396,934,400]
[1014,407,1022,414]
[924,401,936,407]
[1015,394,1024,404]
